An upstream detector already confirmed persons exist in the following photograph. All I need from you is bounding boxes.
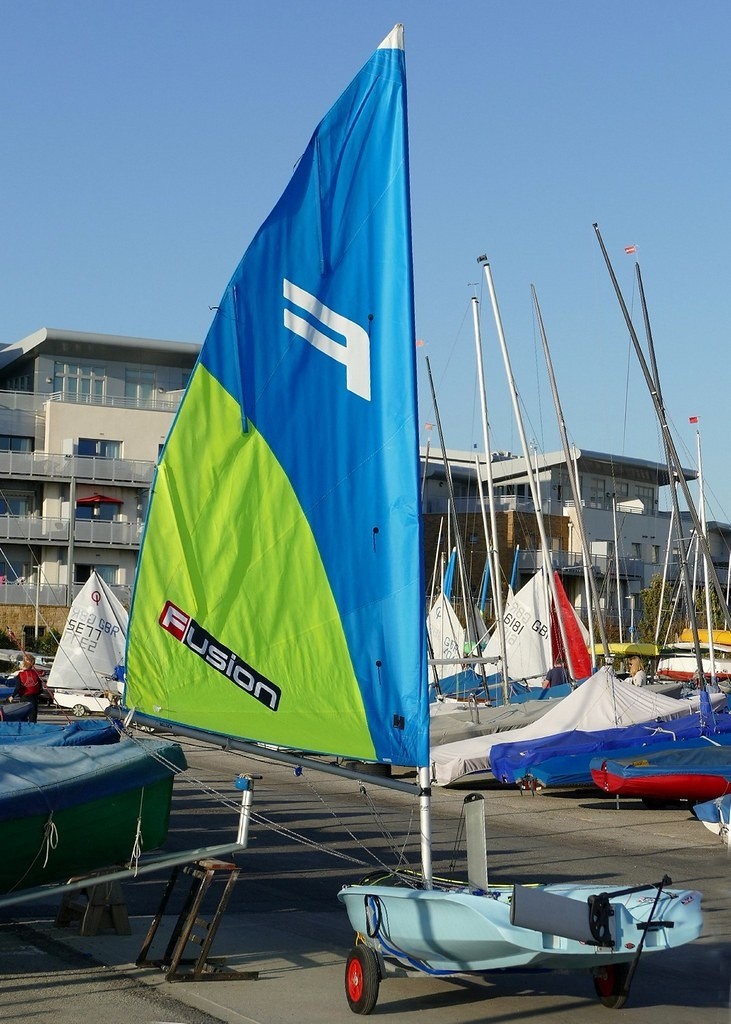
[543,659,569,690]
[8,654,44,723]
[629,657,646,687]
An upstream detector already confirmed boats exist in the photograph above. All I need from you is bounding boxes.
[1,568,128,746]
[0,739,187,896]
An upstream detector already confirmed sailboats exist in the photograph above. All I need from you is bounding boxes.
[47,571,127,711]
[107,23,704,978]
[419,223,731,845]
